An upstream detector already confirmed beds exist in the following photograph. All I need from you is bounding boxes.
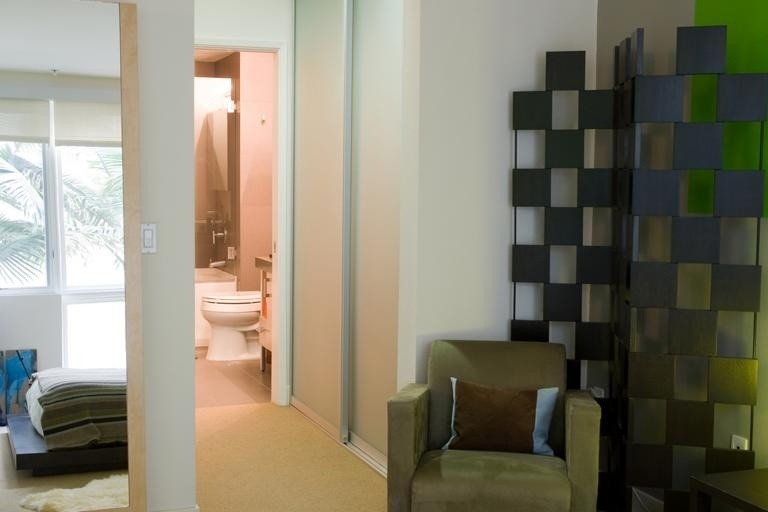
[6,367,128,478]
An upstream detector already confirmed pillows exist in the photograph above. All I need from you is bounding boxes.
[442,377,558,456]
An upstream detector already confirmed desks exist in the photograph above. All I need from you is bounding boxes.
[688,469,768,512]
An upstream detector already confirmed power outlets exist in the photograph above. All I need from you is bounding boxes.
[730,434,749,450]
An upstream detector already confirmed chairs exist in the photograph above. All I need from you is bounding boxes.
[387,340,601,512]
[632,446,754,512]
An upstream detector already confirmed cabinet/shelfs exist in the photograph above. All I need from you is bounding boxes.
[255,255,272,372]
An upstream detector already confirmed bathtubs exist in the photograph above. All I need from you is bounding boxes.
[193,267,237,348]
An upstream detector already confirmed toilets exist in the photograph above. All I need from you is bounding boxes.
[199,290,262,362]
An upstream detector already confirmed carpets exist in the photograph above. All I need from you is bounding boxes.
[19,474,128,512]
